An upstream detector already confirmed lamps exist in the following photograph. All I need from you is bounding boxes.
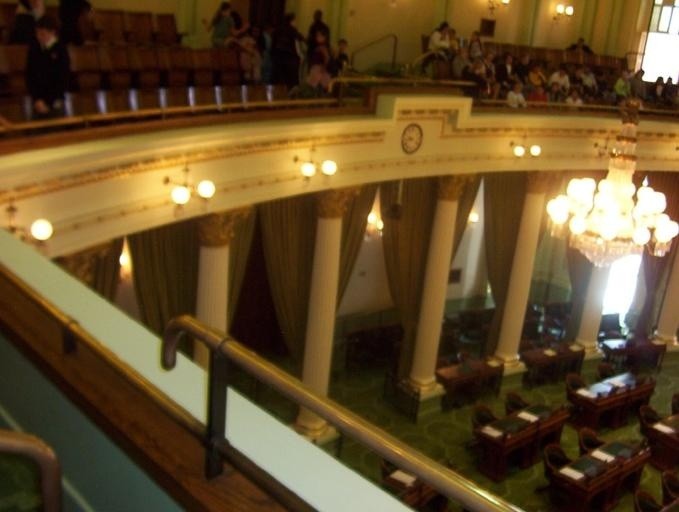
[544,0,679,268]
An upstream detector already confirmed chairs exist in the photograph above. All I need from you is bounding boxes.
[662,469,679,507]
[344,298,668,412]
[470,405,494,431]
[1,1,293,121]
[578,425,605,458]
[420,30,678,121]
[543,443,572,485]
[638,404,661,438]
[671,393,679,416]
[633,489,664,512]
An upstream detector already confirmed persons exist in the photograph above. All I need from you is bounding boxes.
[55,1,97,46]
[208,0,349,93]
[6,0,48,45]
[24,16,79,120]
[427,19,679,110]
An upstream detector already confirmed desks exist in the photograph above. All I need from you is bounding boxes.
[571,367,656,429]
[475,400,572,479]
[645,415,679,471]
[657,498,679,512]
